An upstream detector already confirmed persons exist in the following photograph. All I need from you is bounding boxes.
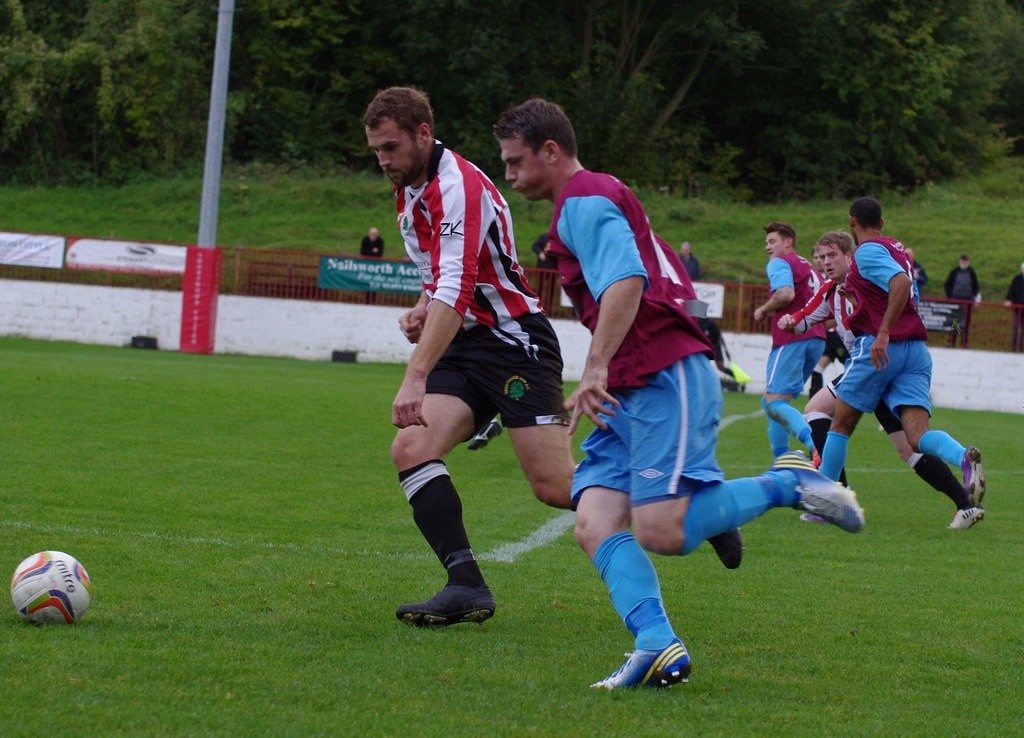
[914,259,928,297]
[531,232,565,318]
[1005,261,1024,354]
[678,241,700,282]
[754,221,827,469]
[360,227,385,305]
[777,196,986,530]
[944,255,981,349]
[489,97,868,693]
[467,418,503,451]
[360,88,745,632]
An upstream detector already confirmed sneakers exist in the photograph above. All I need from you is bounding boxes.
[948,505,984,528]
[590,636,691,688]
[771,450,865,533]
[708,528,744,570]
[396,583,495,626]
[961,446,987,505]
[467,418,503,449]
[799,511,827,524]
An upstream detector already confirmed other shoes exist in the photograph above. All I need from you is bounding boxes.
[811,449,820,464]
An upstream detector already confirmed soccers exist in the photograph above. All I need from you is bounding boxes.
[11,549,92,624]
